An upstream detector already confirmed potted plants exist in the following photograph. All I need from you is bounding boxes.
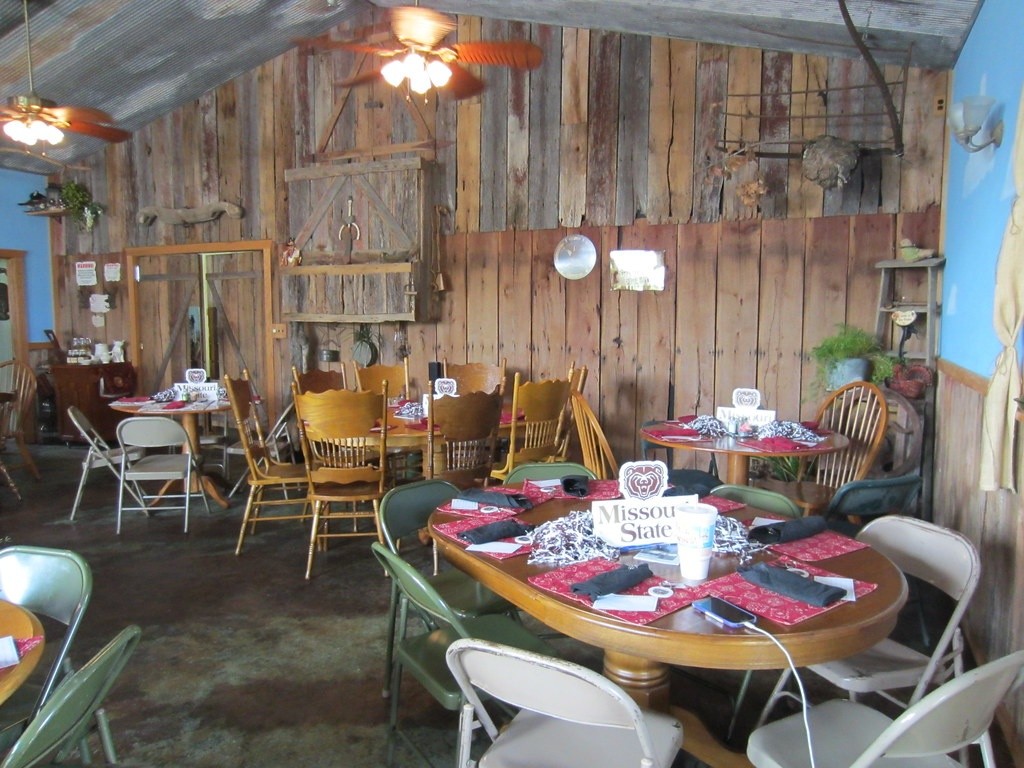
[58,180,103,230]
[799,322,895,404]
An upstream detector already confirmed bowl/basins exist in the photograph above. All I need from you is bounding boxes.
[78,359,93,365]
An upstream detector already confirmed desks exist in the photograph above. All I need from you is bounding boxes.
[299,402,571,489]
[111,394,235,513]
[427,479,909,768]
[641,414,852,496]
[53,361,136,445]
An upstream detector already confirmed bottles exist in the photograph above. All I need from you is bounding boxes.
[728,416,747,434]
[66,337,91,364]
[182,389,189,402]
[189,389,196,402]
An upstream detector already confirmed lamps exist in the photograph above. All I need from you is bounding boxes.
[381,0,452,94]
[4,0,64,145]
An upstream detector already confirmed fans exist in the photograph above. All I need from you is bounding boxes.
[0,0,132,155]
[289,0,542,101]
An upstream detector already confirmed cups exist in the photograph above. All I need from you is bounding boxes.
[674,503,718,582]
[94,342,108,363]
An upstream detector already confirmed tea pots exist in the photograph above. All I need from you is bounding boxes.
[97,351,114,363]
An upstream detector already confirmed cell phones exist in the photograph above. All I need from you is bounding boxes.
[692,596,757,628]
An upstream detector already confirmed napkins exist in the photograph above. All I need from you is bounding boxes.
[119,396,148,402]
[762,436,799,450]
[456,517,533,543]
[163,401,185,409]
[666,481,707,498]
[571,562,651,600]
[458,489,533,509]
[501,410,525,421]
[420,418,439,429]
[651,427,698,439]
[735,559,848,608]
[562,474,588,496]
[748,510,829,544]
[678,415,697,422]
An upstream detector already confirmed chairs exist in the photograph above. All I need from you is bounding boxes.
[68,360,1024,768]
[0,359,46,501]
[0,543,140,768]
